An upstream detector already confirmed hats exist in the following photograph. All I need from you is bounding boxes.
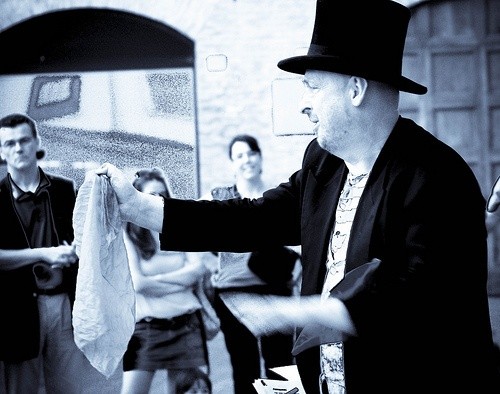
[277,0,428,95]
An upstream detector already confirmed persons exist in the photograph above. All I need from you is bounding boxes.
[87,0,500,394]
[197,134,293,394]
[0,114,84,394]
[122,170,212,394]
[174,367,212,394]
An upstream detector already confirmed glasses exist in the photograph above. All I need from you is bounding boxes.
[134,169,161,179]
